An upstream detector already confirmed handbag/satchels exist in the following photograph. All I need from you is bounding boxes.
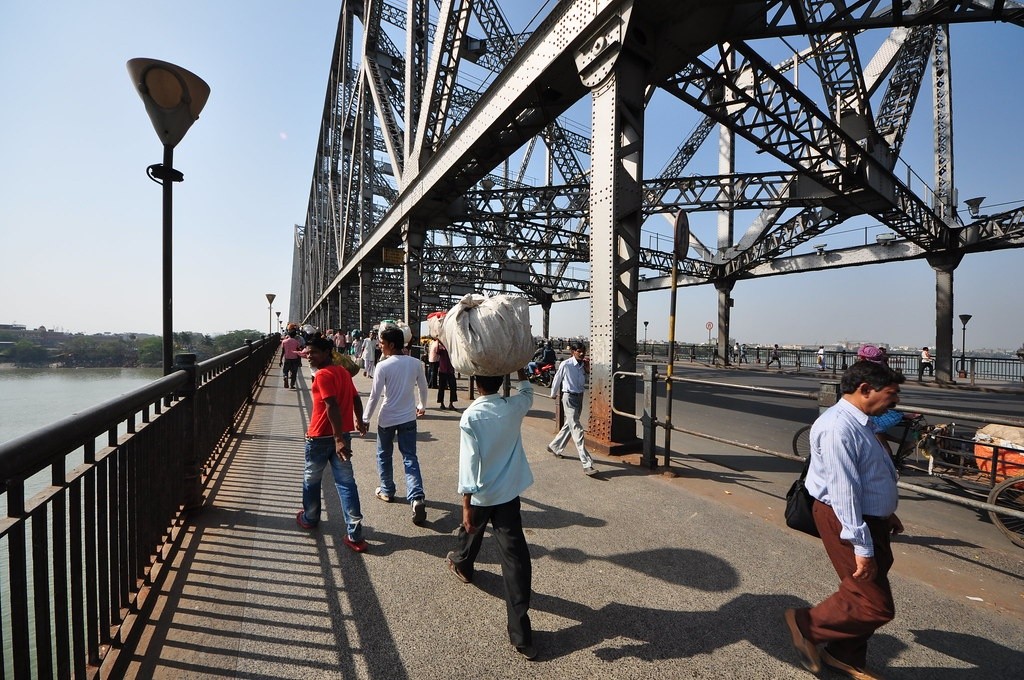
[785,480,821,538]
[334,352,360,377]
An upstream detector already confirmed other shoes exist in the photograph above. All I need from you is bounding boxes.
[449,405,456,411]
[375,486,395,502]
[547,446,563,459]
[412,499,426,523]
[283,378,289,388]
[819,369,824,371]
[289,386,295,389]
[584,467,598,475]
[440,404,445,410]
[784,609,820,673]
[822,647,886,680]
[529,375,536,379]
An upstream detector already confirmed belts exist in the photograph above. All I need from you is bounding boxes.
[568,392,578,396]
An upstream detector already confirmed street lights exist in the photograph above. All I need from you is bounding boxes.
[126,57,212,374]
[644,322,649,351]
[959,314,972,378]
[266,293,277,337]
[275,311,285,336]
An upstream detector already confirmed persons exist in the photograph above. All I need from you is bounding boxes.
[355,328,427,524]
[446,368,538,659]
[674,341,748,364]
[858,345,903,466]
[920,347,934,377]
[815,345,826,371]
[527,342,557,380]
[296,338,368,552]
[547,342,599,476]
[781,360,904,680]
[279,323,459,410]
[766,344,783,370]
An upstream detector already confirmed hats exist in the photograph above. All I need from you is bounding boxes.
[858,344,884,364]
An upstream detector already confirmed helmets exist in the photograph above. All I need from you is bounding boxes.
[537,340,546,347]
[542,341,552,349]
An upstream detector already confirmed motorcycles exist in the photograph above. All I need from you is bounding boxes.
[527,364,555,388]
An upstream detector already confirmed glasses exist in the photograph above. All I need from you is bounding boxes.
[925,349,928,351]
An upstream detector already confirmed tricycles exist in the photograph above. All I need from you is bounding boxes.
[793,412,1024,550]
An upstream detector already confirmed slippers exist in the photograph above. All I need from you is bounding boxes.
[343,534,367,551]
[510,645,537,659]
[447,551,472,583]
[297,511,314,529]
